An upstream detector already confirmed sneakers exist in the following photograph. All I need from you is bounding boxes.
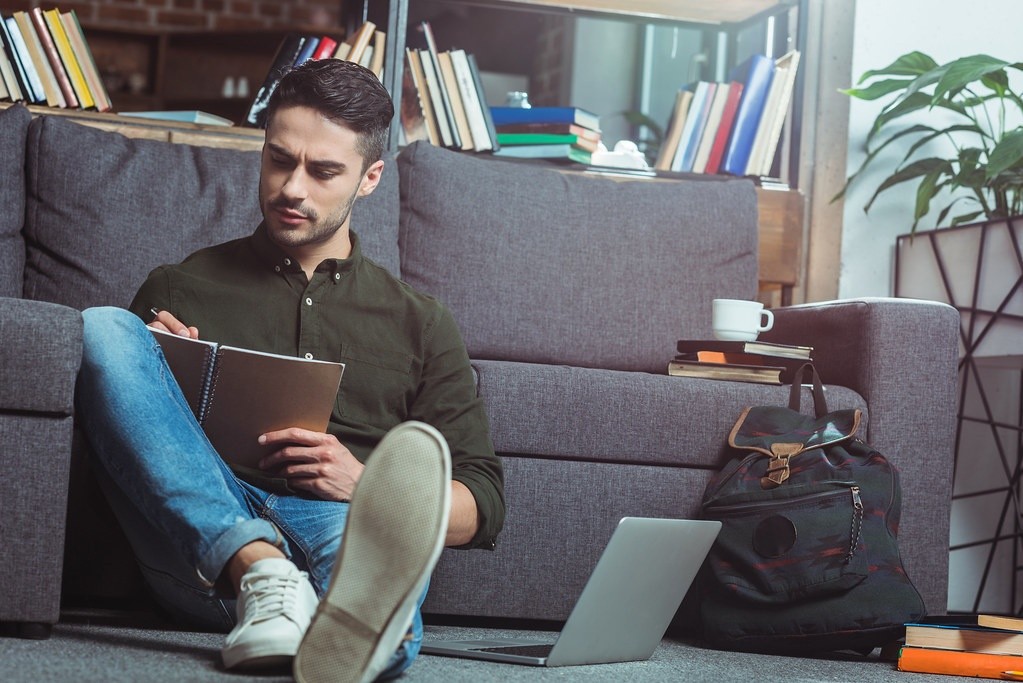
[221,567,318,670]
[293,419,450,683]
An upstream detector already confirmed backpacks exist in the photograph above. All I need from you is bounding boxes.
[682,362,925,651]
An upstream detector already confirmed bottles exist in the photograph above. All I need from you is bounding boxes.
[504,90,532,108]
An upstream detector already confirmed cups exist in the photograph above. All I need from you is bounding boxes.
[712,297,774,342]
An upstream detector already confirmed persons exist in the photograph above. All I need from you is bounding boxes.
[81,60,507,683]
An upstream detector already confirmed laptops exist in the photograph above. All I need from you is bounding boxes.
[420,517,723,667]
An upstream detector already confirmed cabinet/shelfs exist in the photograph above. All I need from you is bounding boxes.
[0,0,858,309]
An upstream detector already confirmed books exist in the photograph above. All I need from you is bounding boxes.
[244,21,386,128]
[146,325,346,478]
[0,7,235,128]
[654,49,803,178]
[897,614,1023,680]
[668,340,814,386]
[406,19,603,166]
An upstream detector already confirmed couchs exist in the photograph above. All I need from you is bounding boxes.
[0,102,962,647]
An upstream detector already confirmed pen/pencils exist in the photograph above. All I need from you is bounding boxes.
[149,306,159,316]
